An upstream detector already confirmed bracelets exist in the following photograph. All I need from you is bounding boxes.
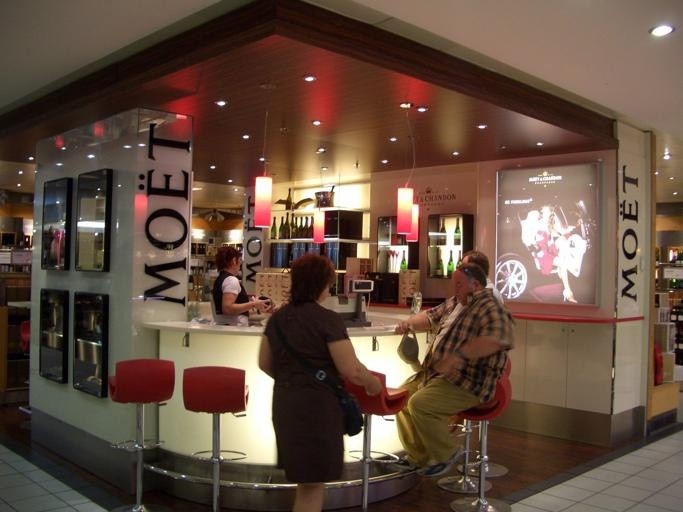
[455,350,470,362]
[252,301,255,307]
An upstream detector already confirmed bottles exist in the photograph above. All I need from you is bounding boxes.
[435,216,464,278]
[270,211,314,239]
[400,249,407,271]
[283,187,292,209]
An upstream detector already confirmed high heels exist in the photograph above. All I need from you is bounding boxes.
[563,289,577,303]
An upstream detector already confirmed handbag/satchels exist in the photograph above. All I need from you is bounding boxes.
[340,394,363,436]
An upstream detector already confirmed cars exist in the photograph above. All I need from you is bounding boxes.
[494,182,599,301]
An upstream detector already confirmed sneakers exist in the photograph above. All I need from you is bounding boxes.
[423,446,464,477]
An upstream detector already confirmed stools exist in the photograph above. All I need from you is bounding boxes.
[433,352,512,512]
[182,366,248,512]
[340,369,411,511]
[106,358,175,512]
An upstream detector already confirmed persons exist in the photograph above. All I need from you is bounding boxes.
[392,263,516,478]
[211,246,271,327]
[462,250,505,306]
[259,253,385,512]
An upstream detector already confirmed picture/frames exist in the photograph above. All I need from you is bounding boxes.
[38,287,68,384]
[492,158,602,308]
[72,291,108,398]
[72,168,113,272]
[41,176,71,272]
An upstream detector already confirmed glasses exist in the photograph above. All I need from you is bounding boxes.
[457,261,471,276]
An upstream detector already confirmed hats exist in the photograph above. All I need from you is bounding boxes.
[397,328,423,372]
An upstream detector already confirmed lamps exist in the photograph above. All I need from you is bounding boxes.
[312,154,325,245]
[252,110,274,228]
[406,204,419,243]
[396,101,416,235]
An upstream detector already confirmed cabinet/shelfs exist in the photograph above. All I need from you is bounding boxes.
[375,213,420,273]
[425,214,474,281]
[268,207,369,295]
